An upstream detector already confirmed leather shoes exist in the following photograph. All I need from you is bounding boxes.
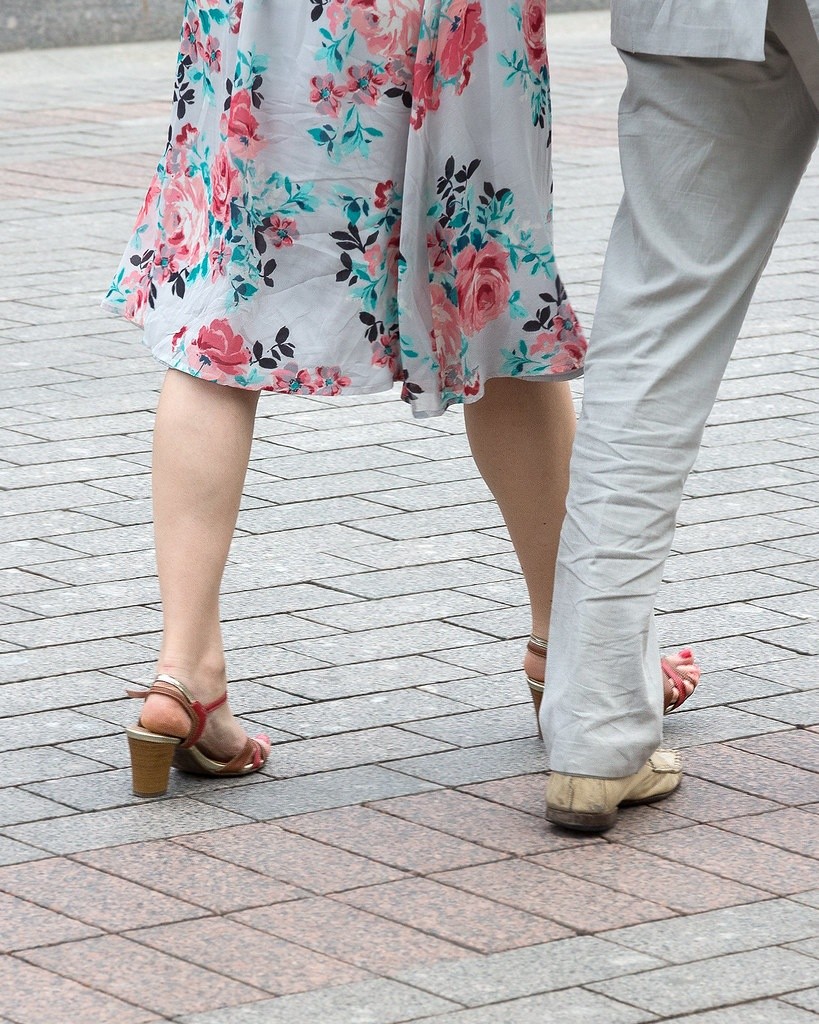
[543,749,684,833]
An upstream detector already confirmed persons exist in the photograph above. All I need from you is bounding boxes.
[534,0,819,832]
[104,1,701,798]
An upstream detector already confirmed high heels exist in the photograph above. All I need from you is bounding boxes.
[523,633,700,741]
[126,674,268,798]
